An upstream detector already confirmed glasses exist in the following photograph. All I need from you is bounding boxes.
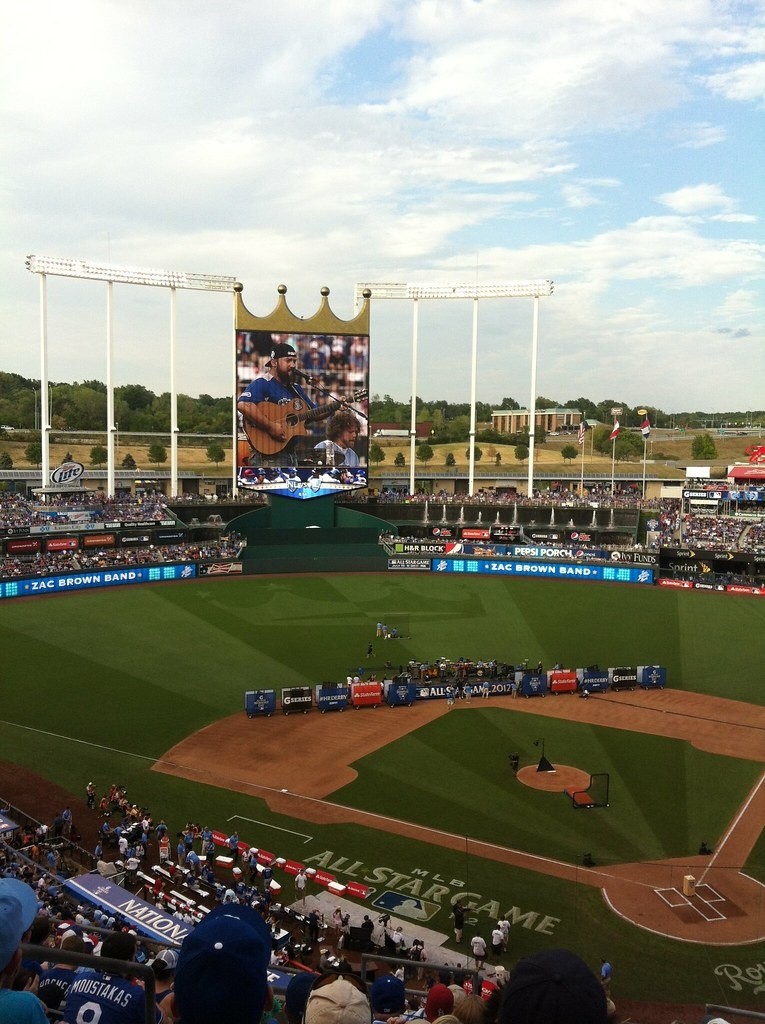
[303,972,373,1024]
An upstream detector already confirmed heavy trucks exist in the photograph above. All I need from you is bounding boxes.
[374,427,410,438]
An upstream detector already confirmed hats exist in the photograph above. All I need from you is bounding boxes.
[497,948,607,1024]
[305,980,372,1024]
[0,878,38,972]
[286,973,317,1015]
[174,903,271,1024]
[155,949,180,970]
[427,984,454,1023]
[447,984,465,1006]
[265,343,296,367]
[371,975,405,1014]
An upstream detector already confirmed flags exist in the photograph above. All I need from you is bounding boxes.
[578,418,585,444]
[641,419,651,439]
[609,419,620,440]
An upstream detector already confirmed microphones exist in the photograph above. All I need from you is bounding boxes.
[291,368,315,380]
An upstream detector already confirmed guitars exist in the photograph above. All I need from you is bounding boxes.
[243,389,368,455]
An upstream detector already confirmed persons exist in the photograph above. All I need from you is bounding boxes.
[366,642,376,658]
[0,488,267,578]
[237,344,345,468]
[0,782,617,1024]
[335,482,765,564]
[238,467,366,485]
[314,413,359,466]
[375,622,397,638]
[346,657,591,711]
[237,331,368,466]
[511,752,519,778]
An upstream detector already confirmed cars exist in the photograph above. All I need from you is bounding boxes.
[737,431,747,437]
[0,425,15,430]
[222,431,232,435]
[546,429,578,436]
[197,430,206,435]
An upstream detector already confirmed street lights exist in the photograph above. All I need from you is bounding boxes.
[116,422,119,464]
[48,384,52,427]
[32,387,38,430]
